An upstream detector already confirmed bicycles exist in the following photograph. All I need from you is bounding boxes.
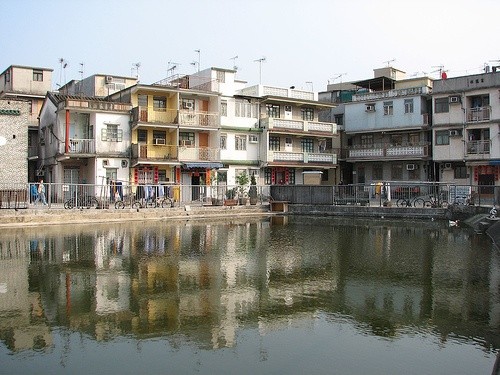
[146,195,173,208]
[396,192,425,208]
[452,196,475,206]
[63,191,99,210]
[114,194,142,209]
[423,195,450,208]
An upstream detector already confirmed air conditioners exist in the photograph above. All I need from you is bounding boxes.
[105,75,113,84]
[406,164,416,171]
[182,140,191,147]
[367,106,373,111]
[445,163,452,168]
[450,97,459,102]
[450,130,458,136]
[154,138,166,146]
[183,102,193,109]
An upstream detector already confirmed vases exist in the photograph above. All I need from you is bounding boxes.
[212,198,223,205]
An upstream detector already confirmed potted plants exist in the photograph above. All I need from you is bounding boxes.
[235,172,249,205]
[224,187,238,205]
[248,176,258,205]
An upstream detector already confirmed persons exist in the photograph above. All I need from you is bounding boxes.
[31,179,47,206]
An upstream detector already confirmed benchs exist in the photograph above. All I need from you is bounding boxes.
[268,201,290,212]
